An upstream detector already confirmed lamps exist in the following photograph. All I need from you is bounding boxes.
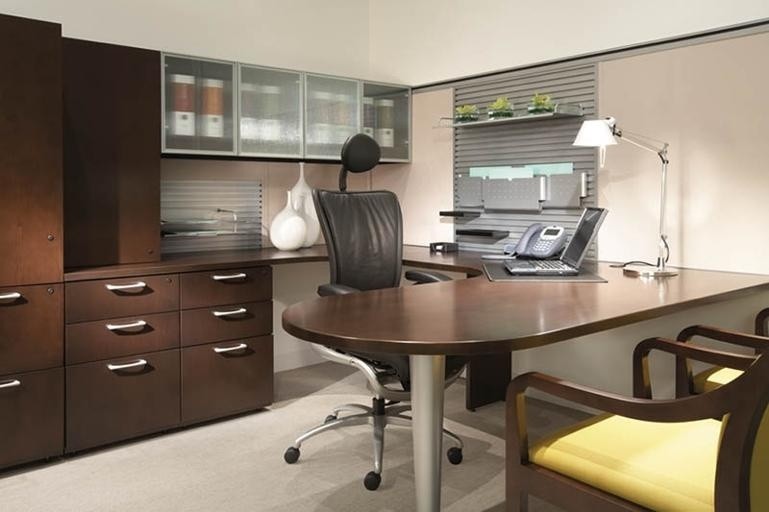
[571,117,678,276]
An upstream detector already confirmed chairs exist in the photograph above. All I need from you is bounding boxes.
[676,311,769,395]
[504,337,769,512]
[283,187,467,490]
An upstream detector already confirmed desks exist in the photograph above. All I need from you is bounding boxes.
[282,243,769,511]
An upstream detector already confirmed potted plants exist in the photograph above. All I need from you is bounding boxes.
[527,90,557,113]
[486,96,514,118]
[454,104,479,123]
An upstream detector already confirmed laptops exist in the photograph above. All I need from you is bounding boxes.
[502,206,608,276]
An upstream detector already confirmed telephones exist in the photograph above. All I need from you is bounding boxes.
[516,222,567,258]
